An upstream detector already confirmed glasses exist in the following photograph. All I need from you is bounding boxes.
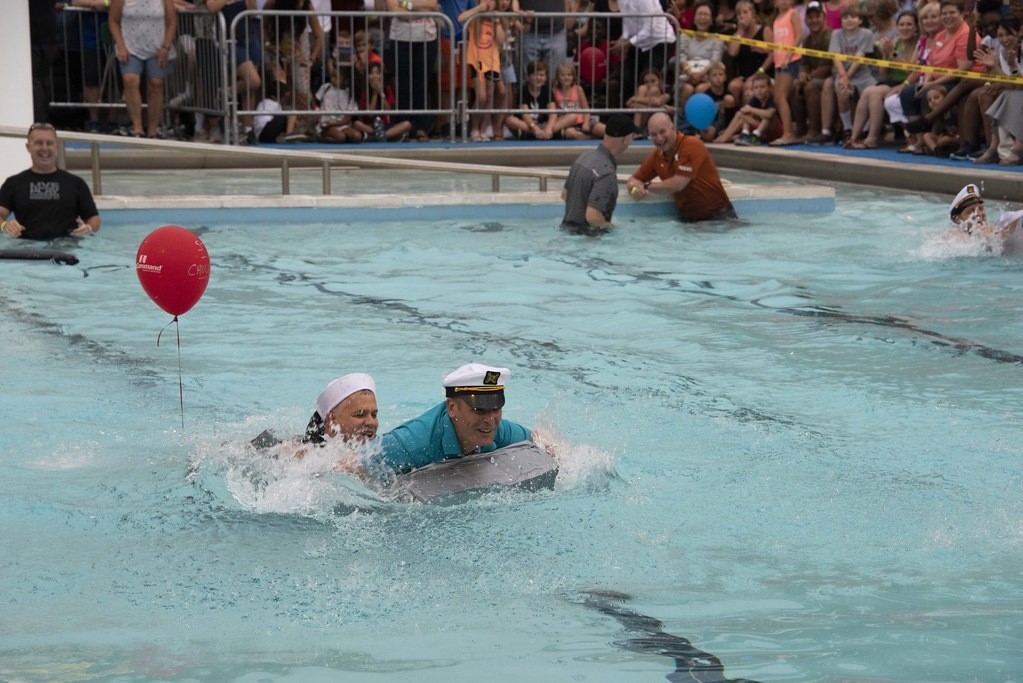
[29,122,57,136]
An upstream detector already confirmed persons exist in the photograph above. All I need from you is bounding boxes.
[255,370,379,489]
[946,183,1023,252]
[0,123,101,242]
[560,117,635,234]
[28,0,1023,166]
[627,112,739,223]
[362,364,558,503]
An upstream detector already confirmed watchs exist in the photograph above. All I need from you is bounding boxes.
[643,180,651,189]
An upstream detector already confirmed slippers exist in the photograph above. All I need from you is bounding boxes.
[843,140,878,149]
[769,137,799,147]
[898,143,917,153]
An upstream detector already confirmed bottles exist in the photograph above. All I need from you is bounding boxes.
[375,117,384,140]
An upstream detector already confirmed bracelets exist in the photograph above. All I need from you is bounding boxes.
[162,43,171,51]
[407,1,412,10]
[983,82,991,86]
[0,221,7,231]
[758,67,765,74]
[903,79,910,86]
[839,70,847,78]
[86,224,94,235]
[628,184,639,196]
[528,121,534,128]
[681,65,691,72]
[401,0,408,8]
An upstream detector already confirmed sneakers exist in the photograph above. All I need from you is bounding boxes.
[950,148,984,161]
[735,133,761,146]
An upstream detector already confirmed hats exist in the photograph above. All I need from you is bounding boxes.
[316,373,375,422]
[949,183,985,220]
[806,1,823,15]
[605,113,634,137]
[441,363,510,411]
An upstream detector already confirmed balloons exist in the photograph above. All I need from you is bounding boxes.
[136,225,210,322]
[580,48,606,85]
[684,93,717,132]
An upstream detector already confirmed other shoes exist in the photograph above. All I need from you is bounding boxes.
[285,132,308,141]
[798,133,834,146]
[1000,149,1023,165]
[244,127,260,145]
[209,127,223,143]
[89,123,119,135]
[471,130,491,142]
[975,148,999,164]
